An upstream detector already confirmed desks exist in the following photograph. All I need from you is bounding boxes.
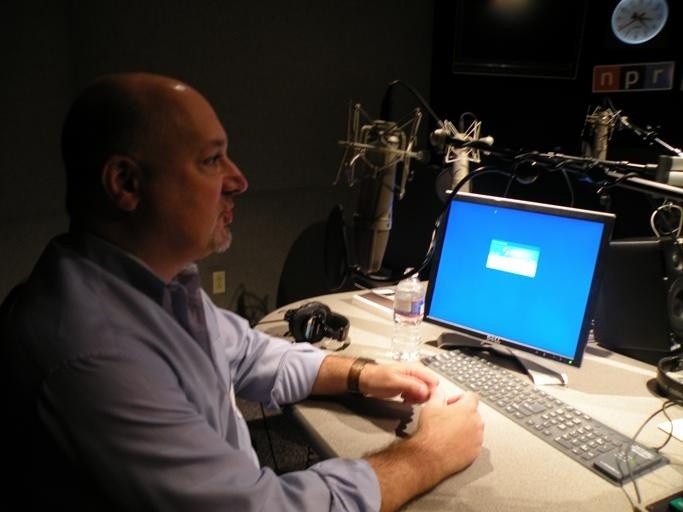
[251,275,682,512]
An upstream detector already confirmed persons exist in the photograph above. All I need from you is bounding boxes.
[0,68,486,511]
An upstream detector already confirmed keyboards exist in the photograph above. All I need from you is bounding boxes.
[420,347,670,487]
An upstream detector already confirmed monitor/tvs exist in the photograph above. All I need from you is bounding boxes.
[422,190,617,385]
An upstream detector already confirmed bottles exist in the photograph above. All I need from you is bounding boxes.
[391,267,425,361]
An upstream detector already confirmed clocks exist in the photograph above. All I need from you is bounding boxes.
[611,0,670,45]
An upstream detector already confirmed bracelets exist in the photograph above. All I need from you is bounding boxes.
[348,355,378,398]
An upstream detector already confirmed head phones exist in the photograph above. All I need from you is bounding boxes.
[284,301,350,346]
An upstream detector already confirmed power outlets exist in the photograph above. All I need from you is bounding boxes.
[212,270,226,296]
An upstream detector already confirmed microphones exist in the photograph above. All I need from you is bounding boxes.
[450,133,470,194]
[354,120,399,275]
[586,110,608,161]
